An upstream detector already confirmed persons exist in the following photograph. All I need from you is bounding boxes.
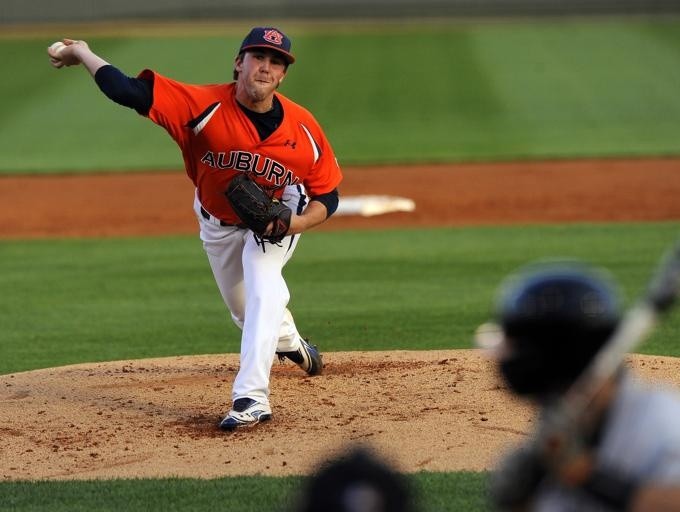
[475,258,678,510]
[45,24,344,432]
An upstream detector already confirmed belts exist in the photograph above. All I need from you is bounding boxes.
[201,206,234,226]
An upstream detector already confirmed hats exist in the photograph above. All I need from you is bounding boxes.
[239,27,296,64]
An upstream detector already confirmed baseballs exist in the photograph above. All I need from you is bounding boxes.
[50,41,67,53]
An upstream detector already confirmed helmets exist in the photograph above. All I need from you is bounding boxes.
[308,455,396,511]
[499,273,623,397]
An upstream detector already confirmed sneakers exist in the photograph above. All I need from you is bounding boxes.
[218,398,273,430]
[276,336,326,376]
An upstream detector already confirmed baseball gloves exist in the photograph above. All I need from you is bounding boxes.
[224,172,292,244]
